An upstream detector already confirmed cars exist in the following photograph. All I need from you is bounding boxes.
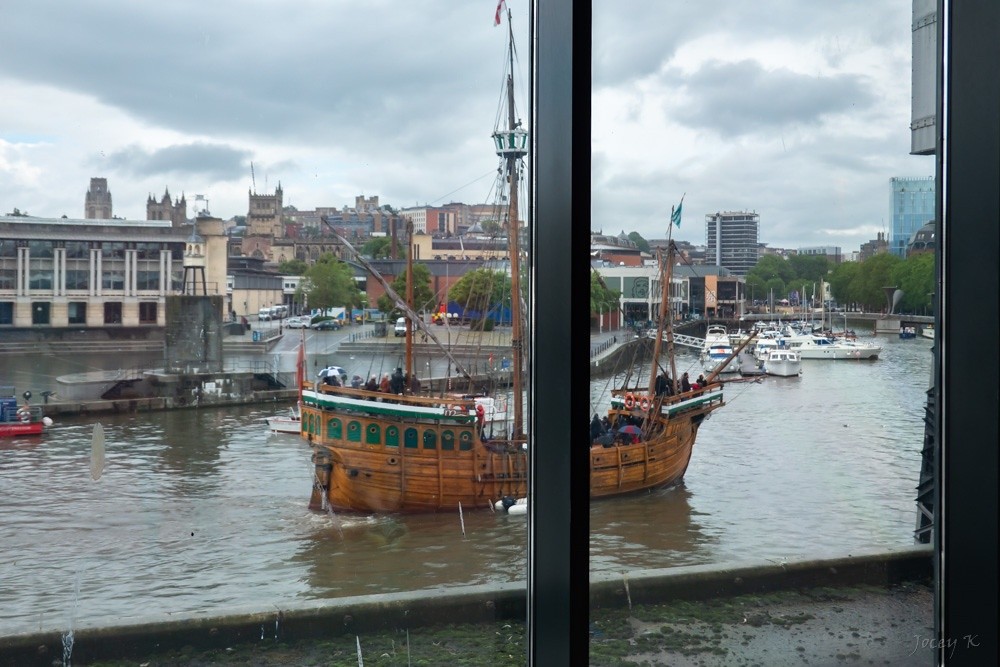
[286,314,313,329]
[310,319,340,331]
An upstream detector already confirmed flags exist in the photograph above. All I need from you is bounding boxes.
[671,202,682,229]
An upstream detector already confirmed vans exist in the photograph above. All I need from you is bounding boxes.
[259,305,288,321]
[394,317,417,336]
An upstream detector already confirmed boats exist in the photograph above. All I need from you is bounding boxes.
[265,414,302,433]
[0,397,44,436]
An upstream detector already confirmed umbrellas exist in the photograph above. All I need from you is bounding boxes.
[617,425,642,435]
[316,365,347,377]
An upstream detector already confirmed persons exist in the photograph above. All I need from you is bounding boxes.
[590,414,657,448]
[476,404,487,440]
[655,371,708,402]
[317,368,423,407]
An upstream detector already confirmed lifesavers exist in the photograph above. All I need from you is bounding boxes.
[19,412,31,424]
[16,407,30,421]
[624,394,635,408]
[449,404,465,421]
[639,396,651,411]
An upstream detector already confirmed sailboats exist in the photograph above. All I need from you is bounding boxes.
[703,280,884,376]
[299,9,724,508]
